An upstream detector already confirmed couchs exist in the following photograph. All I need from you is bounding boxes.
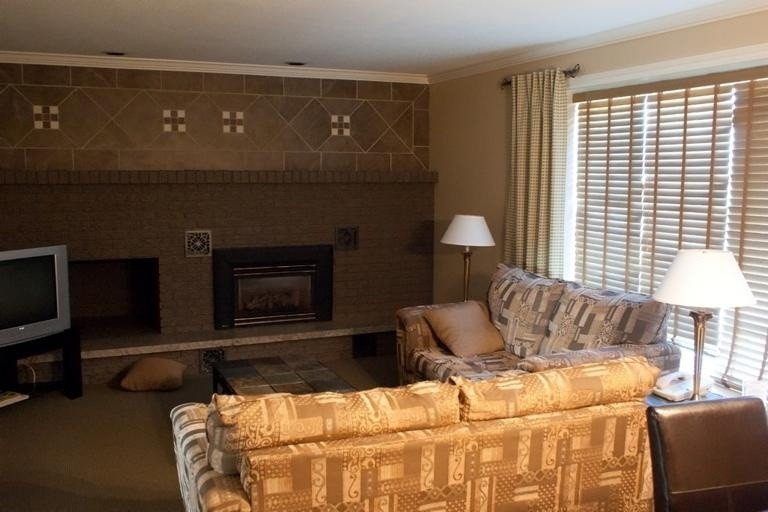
[392,262,686,386]
[165,352,666,511]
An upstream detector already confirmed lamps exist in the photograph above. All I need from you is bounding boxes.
[437,209,498,303]
[650,246,761,403]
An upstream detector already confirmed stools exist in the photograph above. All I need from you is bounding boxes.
[0,323,85,402]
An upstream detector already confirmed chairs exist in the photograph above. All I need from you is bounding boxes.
[640,390,768,512]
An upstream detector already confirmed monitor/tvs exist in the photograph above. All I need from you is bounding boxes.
[0,244,71,348]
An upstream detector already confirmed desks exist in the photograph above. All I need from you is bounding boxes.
[206,356,363,399]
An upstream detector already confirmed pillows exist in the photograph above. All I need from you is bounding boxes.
[483,259,567,358]
[420,296,508,360]
[440,353,663,421]
[537,279,672,358]
[201,379,462,478]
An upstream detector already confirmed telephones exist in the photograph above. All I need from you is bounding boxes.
[651,371,714,402]
